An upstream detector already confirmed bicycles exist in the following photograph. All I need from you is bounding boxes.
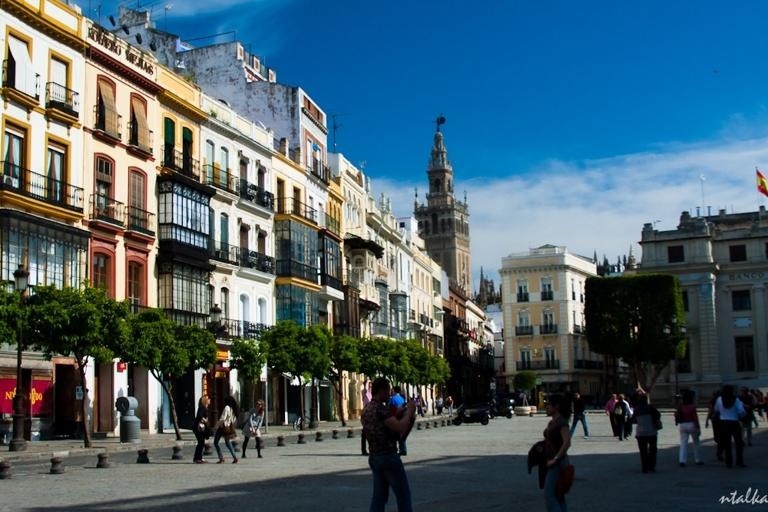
[292,410,312,433]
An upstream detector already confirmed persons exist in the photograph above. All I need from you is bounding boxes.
[536,394,574,512]
[359,377,454,455]
[214,395,240,464]
[192,394,211,464]
[570,385,768,474]
[361,377,415,512]
[241,399,264,457]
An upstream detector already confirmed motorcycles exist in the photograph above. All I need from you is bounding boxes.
[451,399,491,426]
[486,395,515,422]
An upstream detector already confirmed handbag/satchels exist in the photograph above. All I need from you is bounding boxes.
[223,423,235,435]
[249,427,259,434]
[556,464,574,493]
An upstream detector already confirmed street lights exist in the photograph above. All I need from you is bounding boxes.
[10,262,30,453]
[204,298,222,436]
[422,308,446,401]
[663,314,687,409]
